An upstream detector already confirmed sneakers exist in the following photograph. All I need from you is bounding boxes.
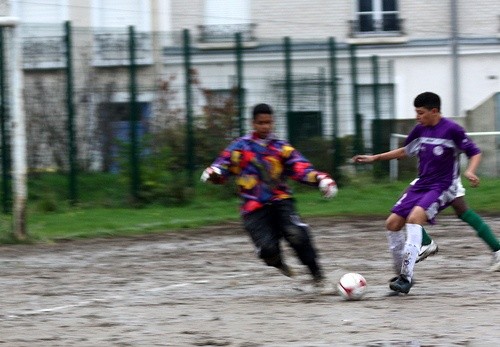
[487,250,500,273]
[389,275,414,293]
[312,270,325,283]
[277,265,291,278]
[411,239,438,263]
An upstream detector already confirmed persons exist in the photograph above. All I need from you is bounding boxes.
[201,104,344,295]
[354,93,482,296]
[414,150,500,273]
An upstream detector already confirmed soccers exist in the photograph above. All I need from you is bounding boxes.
[337,271,368,301]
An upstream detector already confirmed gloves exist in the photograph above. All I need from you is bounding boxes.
[201,164,227,186]
[315,172,339,203]
